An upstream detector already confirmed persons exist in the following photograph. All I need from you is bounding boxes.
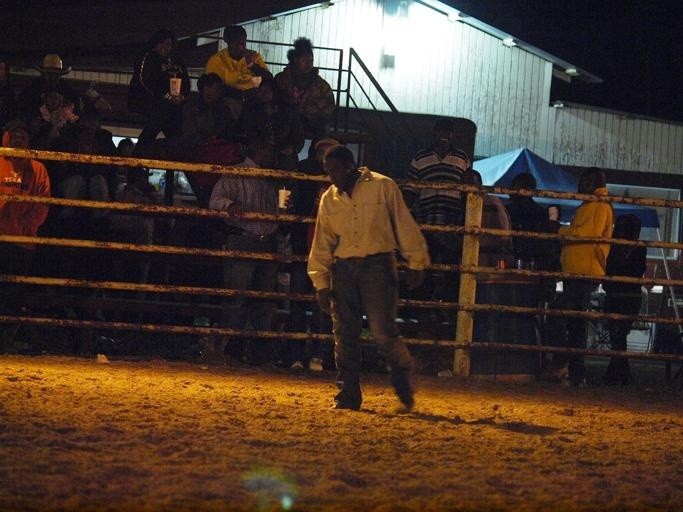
[363,124,649,388]
[1,22,344,373]
[305,143,432,411]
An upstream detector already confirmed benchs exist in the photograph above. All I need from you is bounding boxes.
[0,77,375,153]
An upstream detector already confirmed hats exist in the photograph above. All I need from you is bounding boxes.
[315,139,339,149]
[35,55,71,74]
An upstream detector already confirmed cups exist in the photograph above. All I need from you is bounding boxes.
[278,189,292,209]
[170,78,182,95]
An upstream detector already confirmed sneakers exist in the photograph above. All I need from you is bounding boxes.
[292,359,324,374]
